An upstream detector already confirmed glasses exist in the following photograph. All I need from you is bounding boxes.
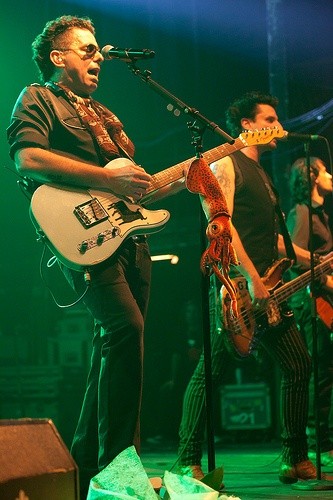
[54,44,101,58]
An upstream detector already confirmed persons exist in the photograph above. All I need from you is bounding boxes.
[280,156,333,466]
[6,15,209,499]
[177,91,333,490]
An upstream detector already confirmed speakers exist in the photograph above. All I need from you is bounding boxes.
[0,418,80,500]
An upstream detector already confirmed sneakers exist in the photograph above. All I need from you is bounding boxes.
[181,466,225,491]
[278,459,333,484]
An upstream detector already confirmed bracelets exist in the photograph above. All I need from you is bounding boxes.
[313,254,320,269]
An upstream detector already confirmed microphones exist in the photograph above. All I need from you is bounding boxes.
[276,130,325,143]
[101,44,155,60]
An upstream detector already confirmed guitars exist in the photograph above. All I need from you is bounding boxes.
[29,127,283,271]
[310,275,333,330]
[219,256,333,360]
